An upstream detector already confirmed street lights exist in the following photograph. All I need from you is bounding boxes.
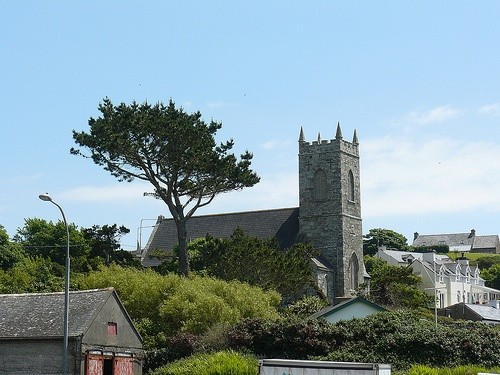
[38,192,70,375]
